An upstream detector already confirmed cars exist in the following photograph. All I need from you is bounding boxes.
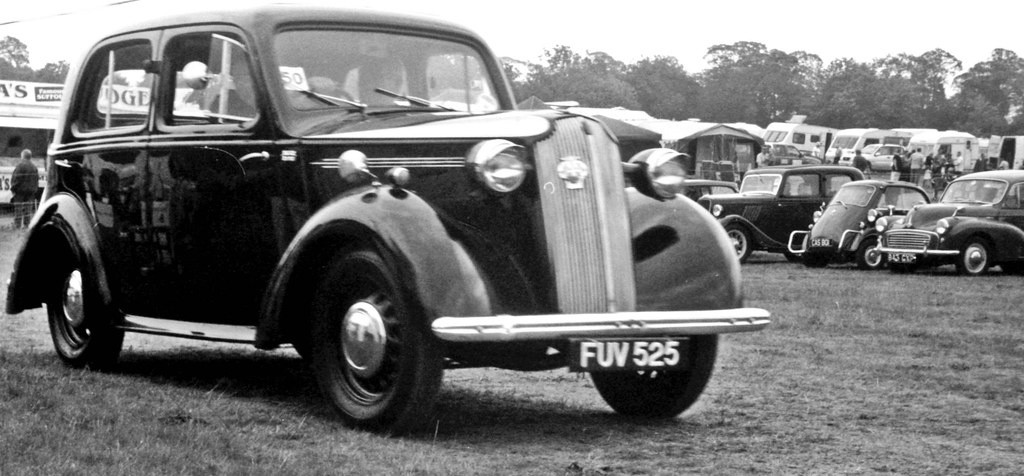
[5,8,772,431]
[788,178,932,270]
[699,164,867,264]
[866,170,1024,276]
[858,143,908,172]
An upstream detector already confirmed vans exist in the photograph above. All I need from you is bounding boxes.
[764,122,831,161]
[906,133,983,173]
[985,134,1024,169]
[823,126,912,164]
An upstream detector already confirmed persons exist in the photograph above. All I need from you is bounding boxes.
[11,149,39,228]
[997,157,1008,170]
[852,149,866,173]
[891,146,963,186]
[974,153,987,173]
[832,144,842,164]
[756,144,770,168]
[814,142,822,158]
[355,58,406,108]
